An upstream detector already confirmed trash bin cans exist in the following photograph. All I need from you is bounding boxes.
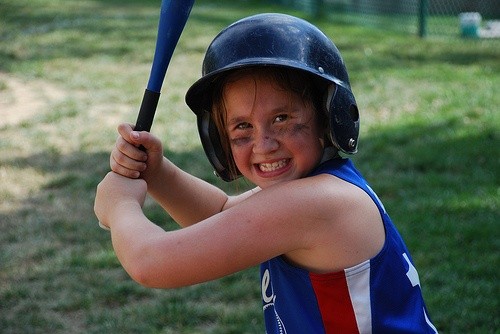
[459,11,481,39]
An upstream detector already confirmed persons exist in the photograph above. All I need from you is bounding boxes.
[92,12,439,333]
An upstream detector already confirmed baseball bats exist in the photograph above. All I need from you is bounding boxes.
[133,0,194,152]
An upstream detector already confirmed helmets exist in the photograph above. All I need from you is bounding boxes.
[184,11,362,181]
